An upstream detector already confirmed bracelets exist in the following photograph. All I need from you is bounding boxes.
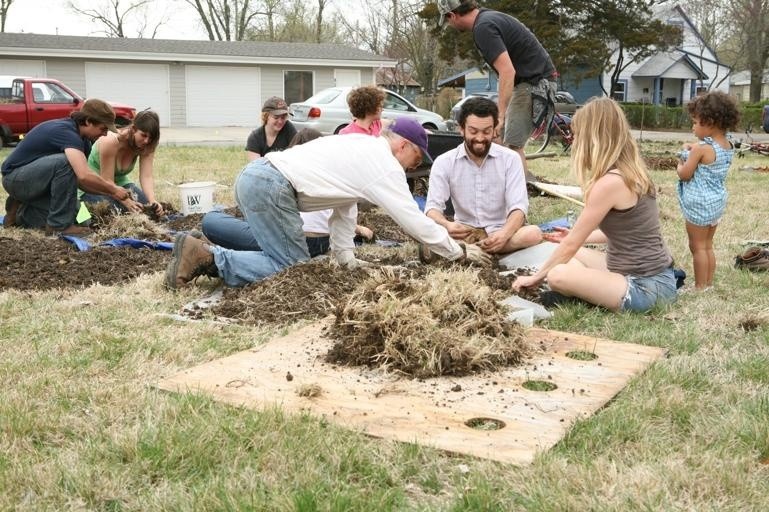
[360,226,364,235]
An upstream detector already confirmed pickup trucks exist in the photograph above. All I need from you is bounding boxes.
[0,77,138,151]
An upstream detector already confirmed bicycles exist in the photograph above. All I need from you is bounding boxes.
[519,86,574,153]
[733,120,769,160]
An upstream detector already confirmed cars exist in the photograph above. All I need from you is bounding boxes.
[283,82,450,139]
[762,105,769,134]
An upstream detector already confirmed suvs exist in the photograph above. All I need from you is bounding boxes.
[450,89,576,133]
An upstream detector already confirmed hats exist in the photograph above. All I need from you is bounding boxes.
[389,117,434,163]
[262,96,289,116]
[436,0,464,26]
[82,100,119,133]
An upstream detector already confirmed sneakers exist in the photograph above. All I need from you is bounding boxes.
[733,246,769,273]
[46,223,92,238]
[4,197,20,227]
[167,229,216,290]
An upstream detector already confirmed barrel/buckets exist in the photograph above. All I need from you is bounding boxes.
[177,181,216,217]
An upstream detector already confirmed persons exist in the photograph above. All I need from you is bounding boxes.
[511,98,678,315]
[338,86,385,137]
[438,0,558,184]
[288,127,373,257]
[676,91,742,292]
[419,97,543,264]
[79,110,165,227]
[163,116,492,291]
[0,99,129,238]
[245,96,297,162]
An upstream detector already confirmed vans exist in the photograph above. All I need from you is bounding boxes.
[0,73,50,102]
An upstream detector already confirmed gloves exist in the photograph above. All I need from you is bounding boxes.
[459,241,493,270]
